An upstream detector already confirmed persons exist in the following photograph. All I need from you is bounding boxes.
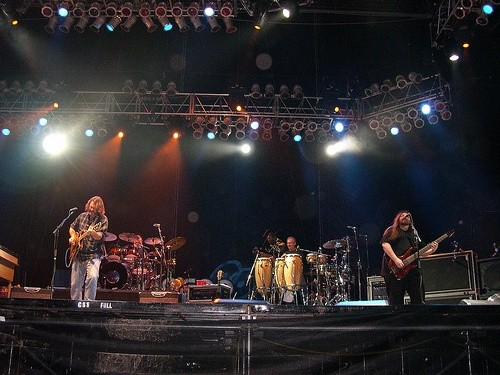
[258,232,307,274]
[70,196,109,301]
[379,210,439,306]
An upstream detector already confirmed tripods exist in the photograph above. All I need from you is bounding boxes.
[328,245,347,306]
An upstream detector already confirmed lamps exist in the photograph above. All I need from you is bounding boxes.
[0,0,300,32]
[0,72,452,141]
[447,0,500,61]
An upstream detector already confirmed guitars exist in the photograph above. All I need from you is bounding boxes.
[70,221,101,259]
[388,228,455,280]
[212,269,228,299]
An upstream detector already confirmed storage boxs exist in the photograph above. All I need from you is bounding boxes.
[418,249,478,301]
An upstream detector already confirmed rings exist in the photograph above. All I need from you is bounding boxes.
[401,263,403,267]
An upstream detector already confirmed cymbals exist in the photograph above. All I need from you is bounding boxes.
[102,232,117,241]
[322,239,347,249]
[118,232,139,242]
[144,237,161,244]
[166,237,186,250]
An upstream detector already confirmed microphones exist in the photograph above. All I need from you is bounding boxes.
[153,224,160,227]
[347,226,357,229]
[70,207,78,211]
[296,245,299,251]
[406,213,410,217]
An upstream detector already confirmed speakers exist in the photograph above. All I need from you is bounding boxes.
[10,288,52,299]
[419,254,472,293]
[476,257,500,300]
[139,292,179,303]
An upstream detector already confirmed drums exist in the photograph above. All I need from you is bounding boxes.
[107,243,121,260]
[147,251,163,261]
[275,257,286,288]
[305,253,318,264]
[254,257,273,292]
[132,258,147,274]
[283,254,303,291]
[318,264,372,302]
[100,262,132,289]
[124,245,139,260]
[316,254,332,266]
